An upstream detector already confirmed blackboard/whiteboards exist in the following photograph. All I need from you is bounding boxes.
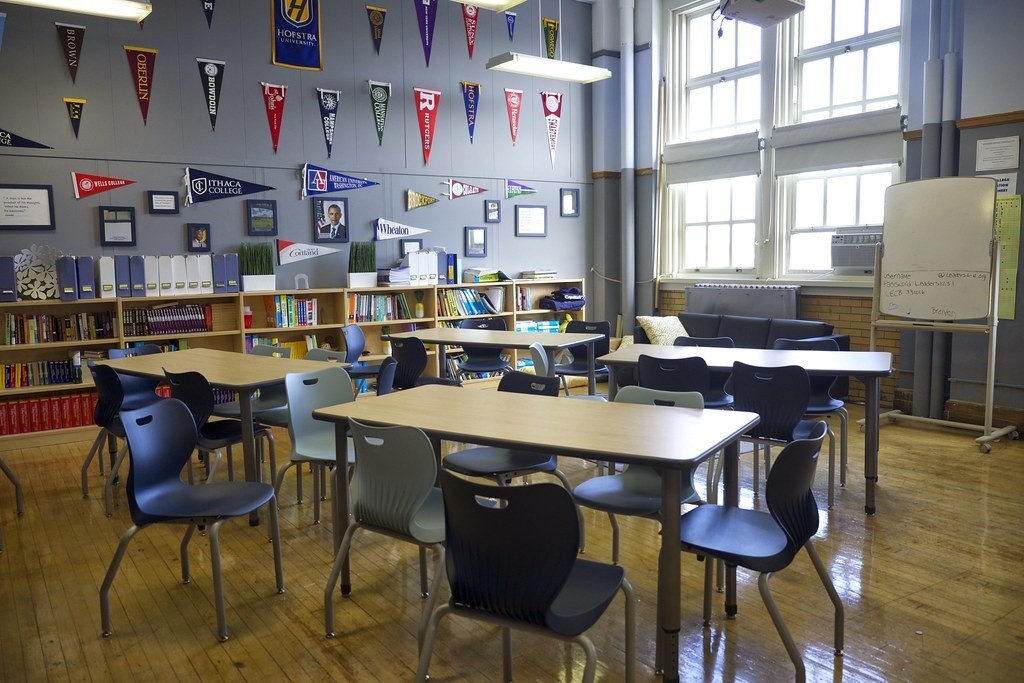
[877,178,996,321]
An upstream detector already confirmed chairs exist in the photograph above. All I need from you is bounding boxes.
[82,321,851,683]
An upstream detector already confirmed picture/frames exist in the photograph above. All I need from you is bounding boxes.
[560,188,580,217]
[464,226,487,257]
[313,196,349,245]
[246,199,278,237]
[99,206,137,246]
[485,200,502,224]
[186,222,212,253]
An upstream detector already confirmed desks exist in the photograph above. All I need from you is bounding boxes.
[311,383,760,683]
[95,348,352,527]
[382,328,605,395]
[596,343,893,516]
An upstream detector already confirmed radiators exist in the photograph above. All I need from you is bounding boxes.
[695,284,802,317]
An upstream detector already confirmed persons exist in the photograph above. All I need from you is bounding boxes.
[193,229,206,247]
[319,204,346,238]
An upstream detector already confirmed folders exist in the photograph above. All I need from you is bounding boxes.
[55,253,241,300]
[401,250,454,285]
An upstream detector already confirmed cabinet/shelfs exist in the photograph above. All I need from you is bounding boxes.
[0,278,584,452]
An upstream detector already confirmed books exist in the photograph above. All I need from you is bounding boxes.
[5,310,118,345]
[517,320,559,333]
[0,350,106,389]
[439,320,463,350]
[347,292,412,323]
[402,323,416,332]
[0,387,236,436]
[125,339,188,357]
[449,353,511,380]
[123,303,207,337]
[264,295,318,328]
[437,287,505,317]
[516,286,532,312]
[523,270,557,278]
[245,334,318,360]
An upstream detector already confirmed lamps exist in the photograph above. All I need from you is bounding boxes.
[485,0,612,85]
[1,0,152,23]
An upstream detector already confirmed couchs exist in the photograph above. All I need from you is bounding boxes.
[634,313,852,399]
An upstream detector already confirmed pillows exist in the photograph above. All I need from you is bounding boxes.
[635,314,691,346]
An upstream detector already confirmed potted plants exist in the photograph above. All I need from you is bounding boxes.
[239,240,276,291]
[347,242,378,289]
